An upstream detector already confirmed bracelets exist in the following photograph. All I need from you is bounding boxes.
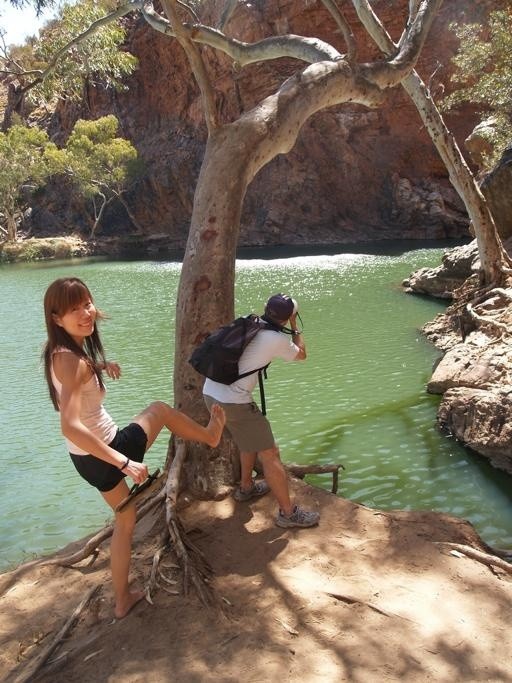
[119,457,130,471]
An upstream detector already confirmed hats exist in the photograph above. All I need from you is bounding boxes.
[265,292,298,320]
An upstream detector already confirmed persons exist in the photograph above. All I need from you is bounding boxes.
[188,294,321,528]
[42,276,227,620]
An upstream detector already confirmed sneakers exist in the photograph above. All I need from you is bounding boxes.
[275,504,320,528]
[233,477,270,501]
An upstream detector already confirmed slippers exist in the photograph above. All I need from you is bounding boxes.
[114,468,167,513]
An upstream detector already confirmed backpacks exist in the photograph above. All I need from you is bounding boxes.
[188,314,282,385]
